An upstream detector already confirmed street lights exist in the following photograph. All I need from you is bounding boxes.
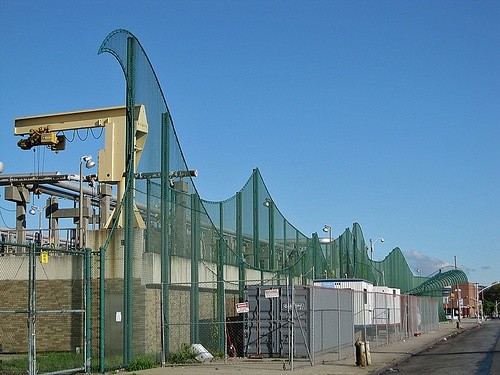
[369,238,384,260]
[323,224,333,273]
[417,268,423,278]
[29,205,43,230]
[262,198,271,209]
[80,154,95,250]
